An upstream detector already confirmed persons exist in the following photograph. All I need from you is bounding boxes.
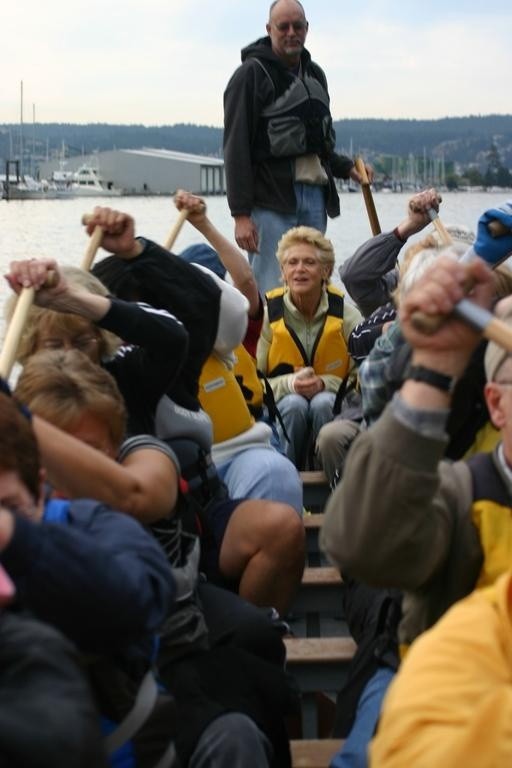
[256,226,364,473]
[220,0,379,309]
[318,189,512,768]
[1,190,306,768]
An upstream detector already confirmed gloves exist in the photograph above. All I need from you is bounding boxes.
[461,202,512,271]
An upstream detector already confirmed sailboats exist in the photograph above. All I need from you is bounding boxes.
[0,80,123,199]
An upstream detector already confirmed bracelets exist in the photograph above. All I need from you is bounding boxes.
[404,362,457,393]
